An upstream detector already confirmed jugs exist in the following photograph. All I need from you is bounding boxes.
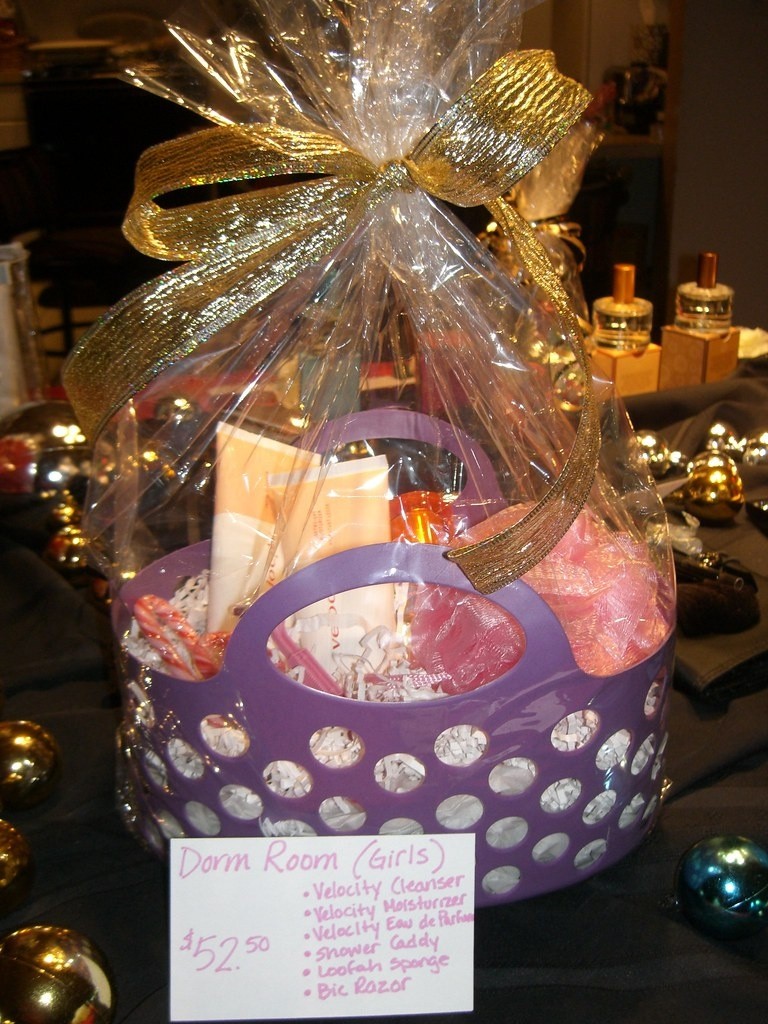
[0,240,45,420]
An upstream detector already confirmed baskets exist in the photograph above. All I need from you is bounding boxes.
[111,407,677,907]
